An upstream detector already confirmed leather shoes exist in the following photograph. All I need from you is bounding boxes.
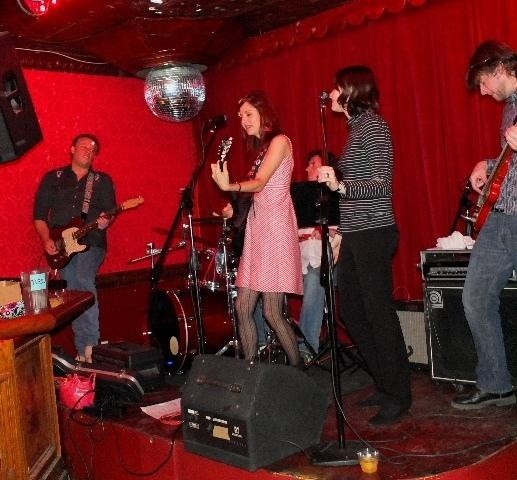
[450,388,515,411]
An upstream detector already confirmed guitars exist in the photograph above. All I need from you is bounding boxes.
[217,137,252,257]
[472,116,517,232]
[46,196,145,270]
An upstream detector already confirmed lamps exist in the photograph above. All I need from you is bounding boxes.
[135,59,208,123]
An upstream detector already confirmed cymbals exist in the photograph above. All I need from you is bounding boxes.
[190,216,231,224]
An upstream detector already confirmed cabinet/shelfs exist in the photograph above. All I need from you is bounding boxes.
[0,332,68,480]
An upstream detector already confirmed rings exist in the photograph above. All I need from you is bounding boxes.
[326,173,329,178]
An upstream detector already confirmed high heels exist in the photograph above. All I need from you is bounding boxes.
[358,390,384,405]
[368,406,407,425]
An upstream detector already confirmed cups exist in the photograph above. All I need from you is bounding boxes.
[356,448,380,474]
[21,269,49,314]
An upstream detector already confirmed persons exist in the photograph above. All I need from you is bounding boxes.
[253,150,343,363]
[33,133,118,363]
[211,91,305,370]
[449,39,517,410]
[317,64,413,427]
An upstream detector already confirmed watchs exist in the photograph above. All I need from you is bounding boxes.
[335,182,342,191]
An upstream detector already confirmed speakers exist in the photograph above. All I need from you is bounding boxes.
[181,354,327,472]
[425,279,515,384]
[0,29,44,165]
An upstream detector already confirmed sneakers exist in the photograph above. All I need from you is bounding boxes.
[298,350,313,365]
[257,345,268,358]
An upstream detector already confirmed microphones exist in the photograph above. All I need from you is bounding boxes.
[203,113,231,124]
[316,90,329,106]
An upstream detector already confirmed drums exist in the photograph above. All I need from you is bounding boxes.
[197,247,236,290]
[149,289,234,376]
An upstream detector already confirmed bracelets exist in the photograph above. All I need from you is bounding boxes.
[237,182,241,192]
